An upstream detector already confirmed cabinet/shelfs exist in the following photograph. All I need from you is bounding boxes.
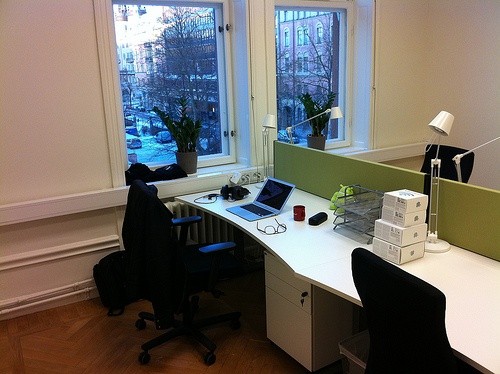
[263,251,353,372]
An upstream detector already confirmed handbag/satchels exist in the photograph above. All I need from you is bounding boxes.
[92,250,142,317]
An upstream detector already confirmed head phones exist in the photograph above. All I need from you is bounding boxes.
[222,185,250,201]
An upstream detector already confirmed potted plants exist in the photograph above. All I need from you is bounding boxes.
[300,92,337,151]
[152,97,204,175]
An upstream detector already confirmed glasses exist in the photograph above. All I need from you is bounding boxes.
[256,219,287,235]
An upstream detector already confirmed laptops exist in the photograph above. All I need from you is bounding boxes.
[225,177,295,222]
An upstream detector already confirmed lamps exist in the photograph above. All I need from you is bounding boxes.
[285,105,343,145]
[424,111,455,254]
[256,114,276,188]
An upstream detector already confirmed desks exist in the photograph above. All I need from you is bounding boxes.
[175,181,500,374]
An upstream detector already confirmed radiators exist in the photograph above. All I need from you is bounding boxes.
[163,201,236,255]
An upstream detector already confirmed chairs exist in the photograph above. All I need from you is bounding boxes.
[350,246,464,374]
[121,180,241,367]
[420,143,474,223]
